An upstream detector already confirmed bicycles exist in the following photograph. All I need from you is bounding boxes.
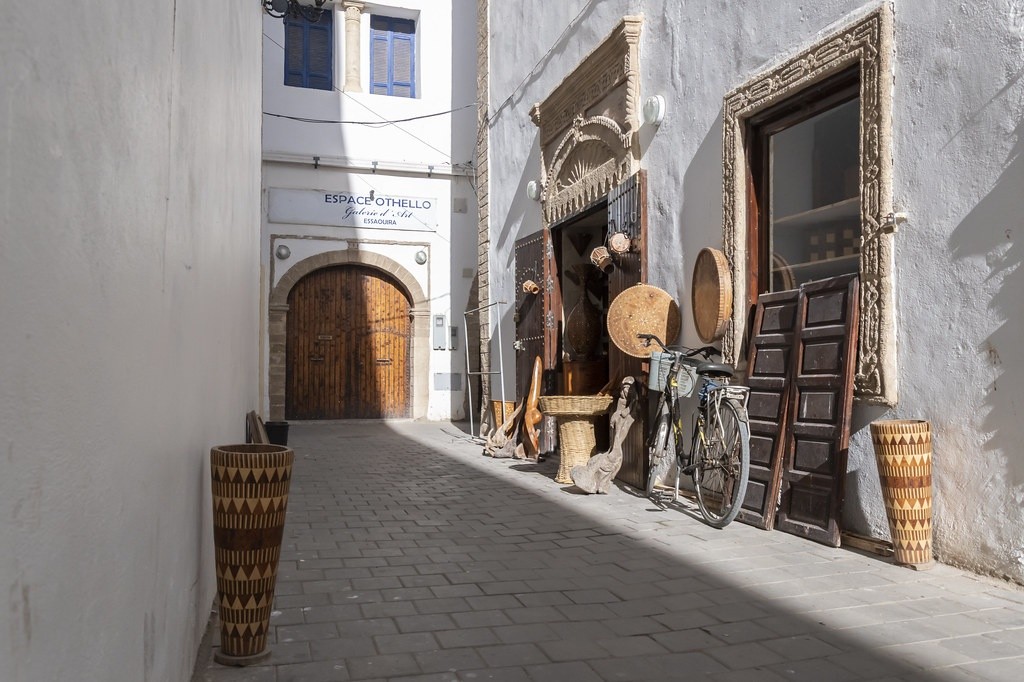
[635,333,751,528]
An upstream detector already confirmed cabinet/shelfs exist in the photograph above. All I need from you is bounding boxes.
[773,195,862,275]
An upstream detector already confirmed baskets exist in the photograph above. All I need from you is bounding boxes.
[537,395,614,416]
[647,351,698,399]
[607,284,681,358]
[691,247,734,344]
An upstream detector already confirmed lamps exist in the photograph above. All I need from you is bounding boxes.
[527,180,540,201]
[643,95,665,125]
